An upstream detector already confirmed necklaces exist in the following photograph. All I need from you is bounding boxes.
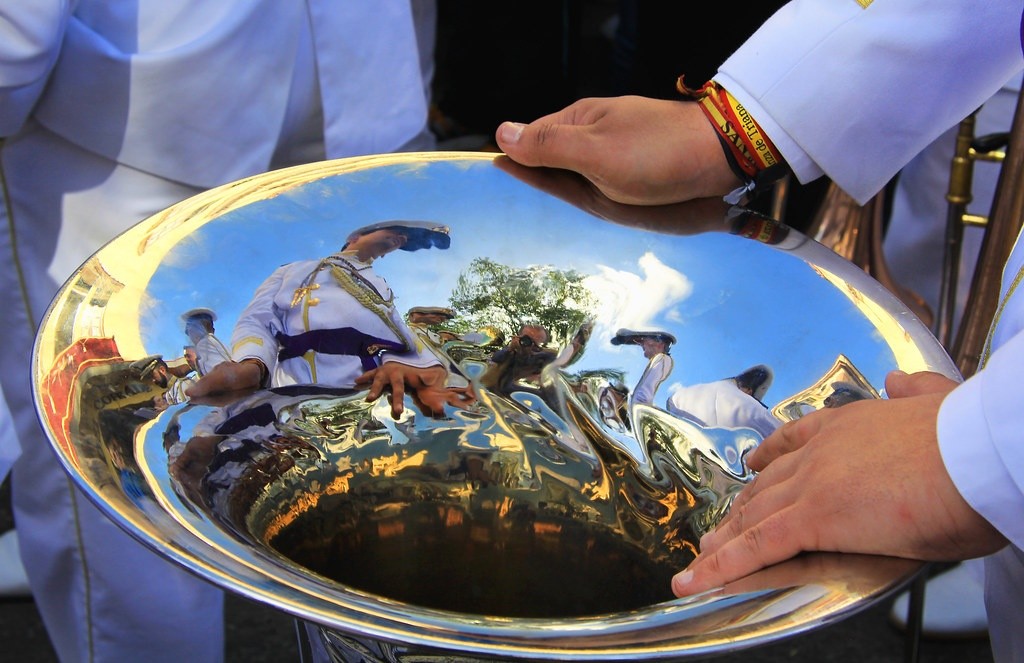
[418,327,429,338]
[328,255,373,279]
[168,383,174,400]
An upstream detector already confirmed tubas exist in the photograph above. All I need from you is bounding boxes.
[29,78,1017,662]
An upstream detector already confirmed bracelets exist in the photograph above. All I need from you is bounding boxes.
[678,75,778,183]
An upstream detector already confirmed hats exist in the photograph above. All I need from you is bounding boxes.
[129,354,163,380]
[181,308,217,321]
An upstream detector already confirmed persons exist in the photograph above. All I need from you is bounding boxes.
[123,220,880,537]
[493,0,1024,663]
[0,0,440,663]
